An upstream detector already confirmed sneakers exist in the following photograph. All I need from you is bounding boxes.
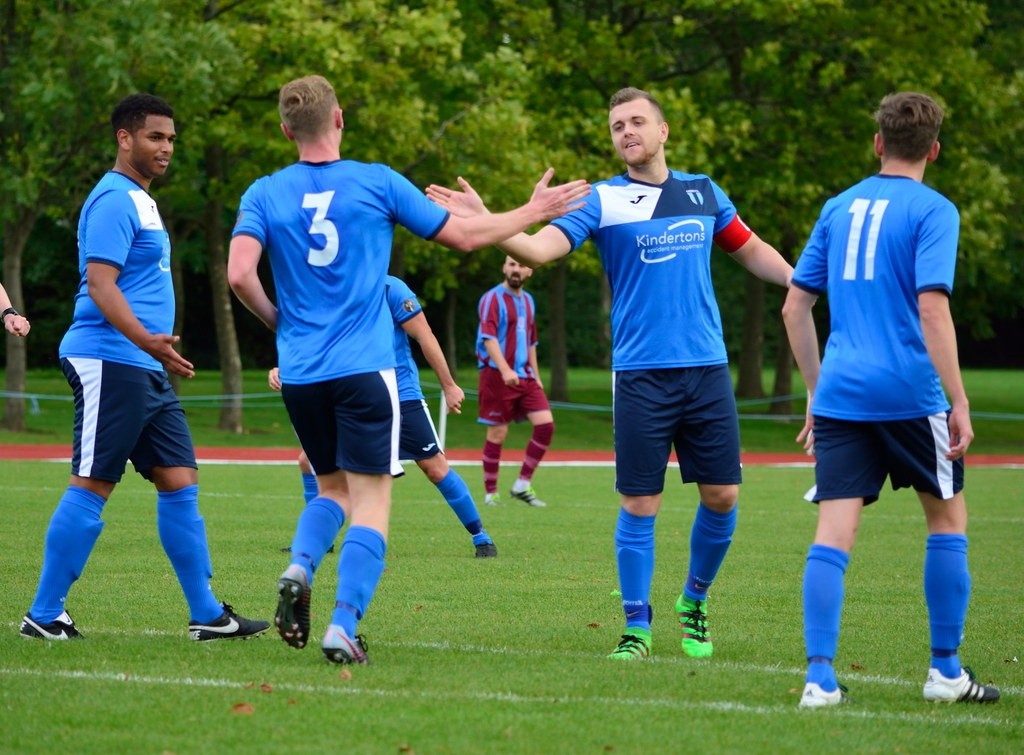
[922,667,1000,706]
[20,609,85,641]
[485,493,505,506]
[609,628,653,663]
[284,543,335,556]
[510,480,548,507]
[321,624,374,665]
[473,542,498,559]
[676,594,714,660]
[798,683,856,708]
[273,564,311,648]
[188,602,270,645]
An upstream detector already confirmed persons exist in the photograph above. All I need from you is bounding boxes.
[0,284,31,338]
[422,87,796,661]
[226,73,593,665]
[16,92,271,643]
[265,271,498,559]
[475,253,557,508]
[781,92,1001,711]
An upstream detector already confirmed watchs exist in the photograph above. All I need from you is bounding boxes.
[2,307,18,320]
